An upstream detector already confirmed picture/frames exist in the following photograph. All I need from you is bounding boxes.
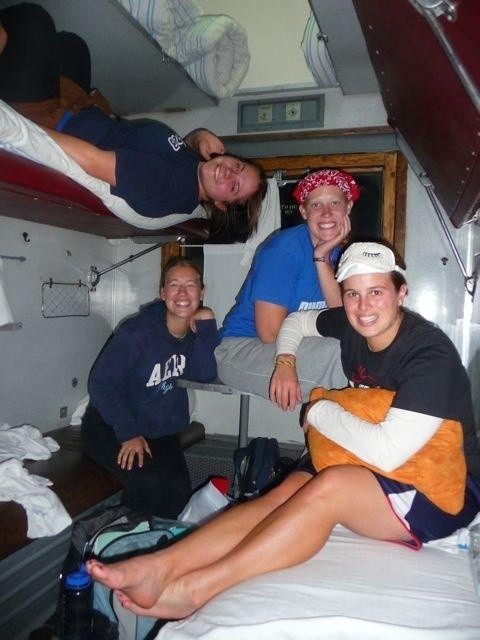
[156,150,405,335]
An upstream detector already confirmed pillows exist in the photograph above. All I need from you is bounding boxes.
[306,384,468,515]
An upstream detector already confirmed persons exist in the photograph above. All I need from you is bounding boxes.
[85,237,480,621]
[214,167,360,414]
[0,0,268,243]
[80,255,218,520]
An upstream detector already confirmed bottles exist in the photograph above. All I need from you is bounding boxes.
[53,562,95,640]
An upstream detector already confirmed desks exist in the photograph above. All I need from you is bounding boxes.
[177,372,258,500]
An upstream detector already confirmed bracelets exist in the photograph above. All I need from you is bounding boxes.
[274,358,295,369]
[313,254,330,263]
